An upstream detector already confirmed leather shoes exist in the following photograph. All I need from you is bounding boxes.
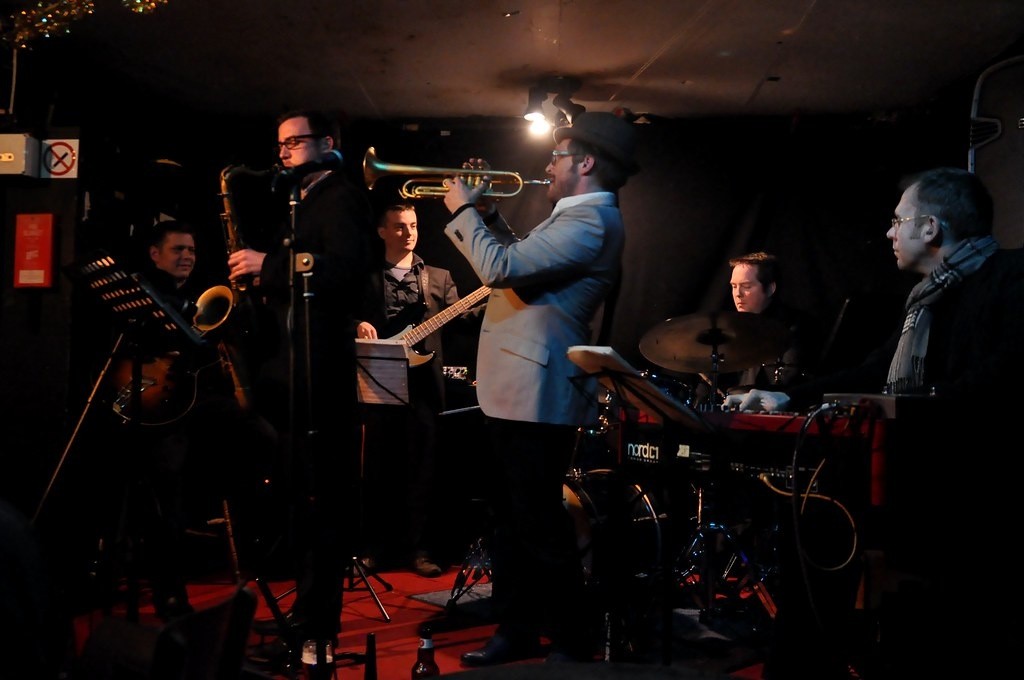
[462,632,542,667]
[253,605,341,639]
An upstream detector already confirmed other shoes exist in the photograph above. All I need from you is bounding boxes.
[414,556,443,576]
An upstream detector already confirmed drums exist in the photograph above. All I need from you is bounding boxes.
[562,467,662,623]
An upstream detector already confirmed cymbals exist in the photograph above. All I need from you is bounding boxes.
[597,385,612,403]
[640,311,794,373]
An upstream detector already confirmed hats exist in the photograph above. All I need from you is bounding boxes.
[552,112,639,170]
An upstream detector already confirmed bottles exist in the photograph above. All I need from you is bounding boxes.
[411,628,439,680]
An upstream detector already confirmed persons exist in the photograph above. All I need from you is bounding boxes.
[354,203,487,577]
[443,112,643,666]
[722,252,799,393]
[151,220,197,626]
[872,168,1024,680]
[227,107,367,660]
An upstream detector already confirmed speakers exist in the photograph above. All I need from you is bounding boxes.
[967,54,1024,249]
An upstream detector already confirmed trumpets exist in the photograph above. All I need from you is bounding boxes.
[362,146,551,201]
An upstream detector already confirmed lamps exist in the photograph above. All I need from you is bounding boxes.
[523,89,586,140]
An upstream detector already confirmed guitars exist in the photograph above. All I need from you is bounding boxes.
[375,285,493,366]
[105,338,223,428]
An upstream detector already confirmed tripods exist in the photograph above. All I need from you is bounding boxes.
[671,344,778,630]
[275,356,411,621]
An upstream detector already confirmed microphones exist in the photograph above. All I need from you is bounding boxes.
[272,149,344,190]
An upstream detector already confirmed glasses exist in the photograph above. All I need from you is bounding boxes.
[552,149,597,165]
[891,215,951,229]
[273,134,323,149]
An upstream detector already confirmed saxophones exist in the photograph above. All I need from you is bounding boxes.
[188,163,288,410]
